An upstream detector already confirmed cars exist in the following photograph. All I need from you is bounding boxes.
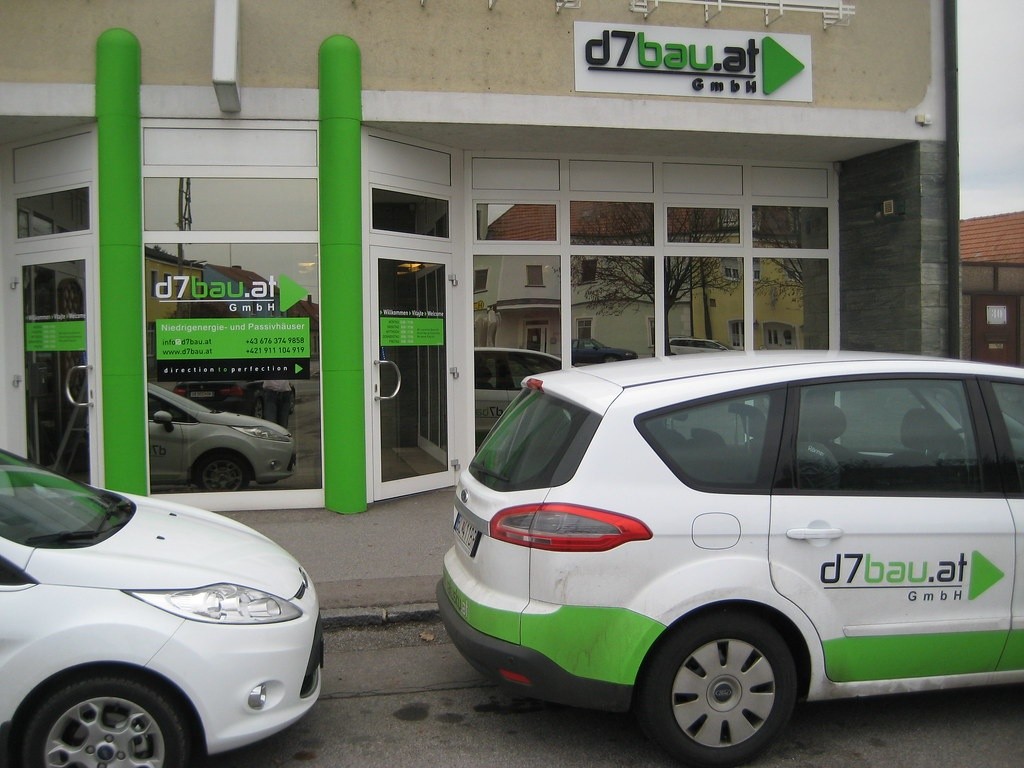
[171,374,298,416]
[472,347,581,441]
[553,337,641,371]
[146,383,296,492]
[432,350,1024,768]
[0,446,328,768]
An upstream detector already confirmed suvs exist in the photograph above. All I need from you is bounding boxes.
[653,336,733,358]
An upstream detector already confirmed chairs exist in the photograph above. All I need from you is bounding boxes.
[881,411,947,497]
[687,428,730,484]
[799,406,873,496]
